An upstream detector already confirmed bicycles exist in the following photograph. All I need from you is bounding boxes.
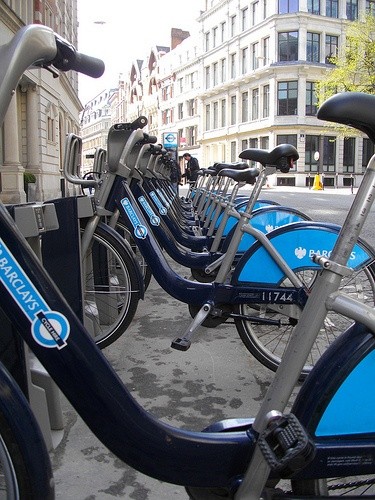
[0,23,375,500]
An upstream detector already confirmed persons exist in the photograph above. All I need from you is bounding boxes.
[180,153,200,195]
